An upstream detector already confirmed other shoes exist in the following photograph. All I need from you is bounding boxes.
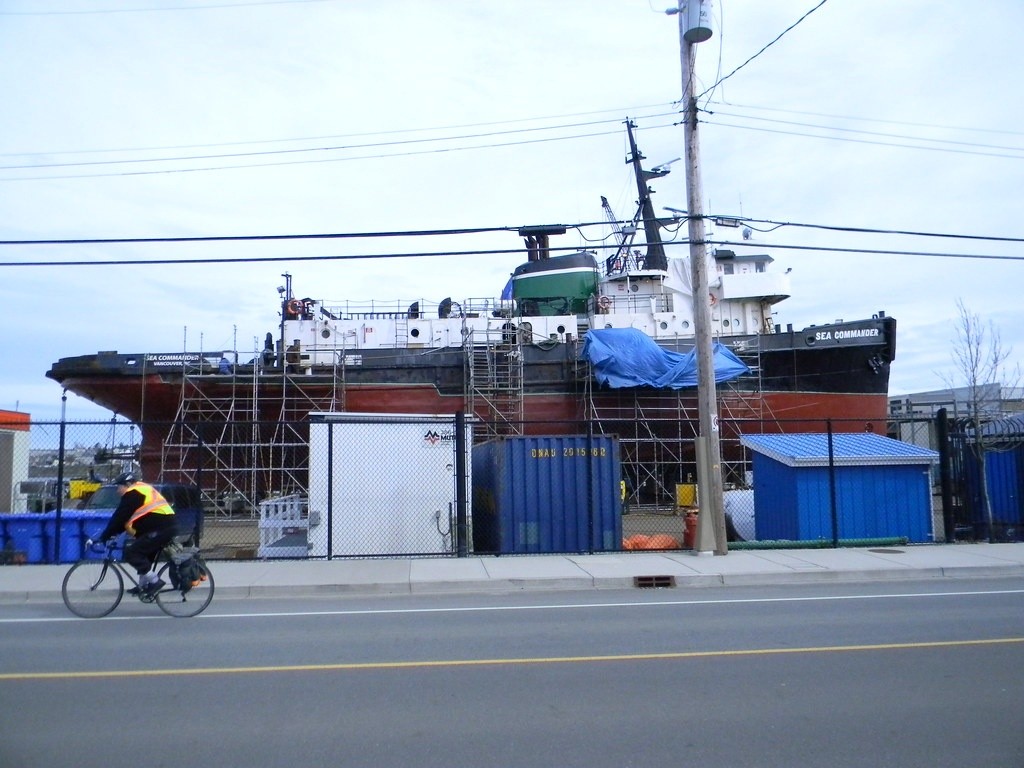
[127,586,141,594]
[145,579,167,599]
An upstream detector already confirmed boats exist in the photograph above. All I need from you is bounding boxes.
[44,116,897,484]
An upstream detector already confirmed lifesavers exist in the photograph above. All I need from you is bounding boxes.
[598,296,611,309]
[288,299,304,315]
[709,292,716,306]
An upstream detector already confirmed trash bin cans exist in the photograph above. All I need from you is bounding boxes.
[5,514,44,564]
[76,510,127,561]
[0,514,7,554]
[38,509,86,562]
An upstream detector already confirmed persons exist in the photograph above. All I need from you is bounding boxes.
[90,472,176,598]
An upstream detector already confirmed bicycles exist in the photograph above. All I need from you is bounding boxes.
[61,529,218,618]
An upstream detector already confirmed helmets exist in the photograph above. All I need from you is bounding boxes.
[114,472,137,486]
